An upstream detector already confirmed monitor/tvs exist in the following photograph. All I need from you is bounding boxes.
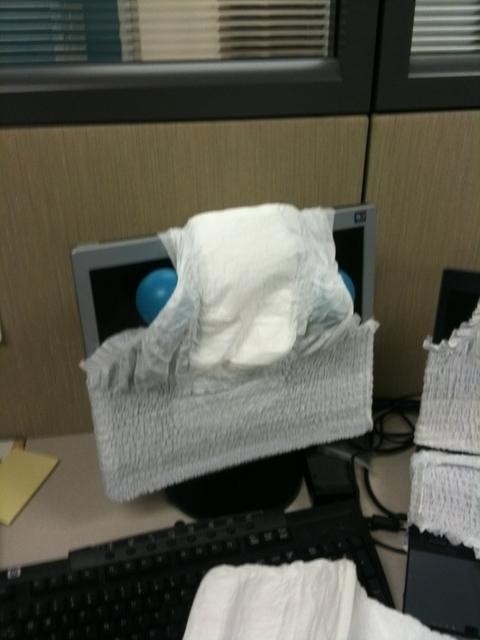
[69,203,376,518]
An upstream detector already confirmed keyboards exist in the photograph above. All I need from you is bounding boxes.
[0,501,395,640]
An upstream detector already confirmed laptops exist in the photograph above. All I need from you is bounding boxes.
[402,270,480,639]
